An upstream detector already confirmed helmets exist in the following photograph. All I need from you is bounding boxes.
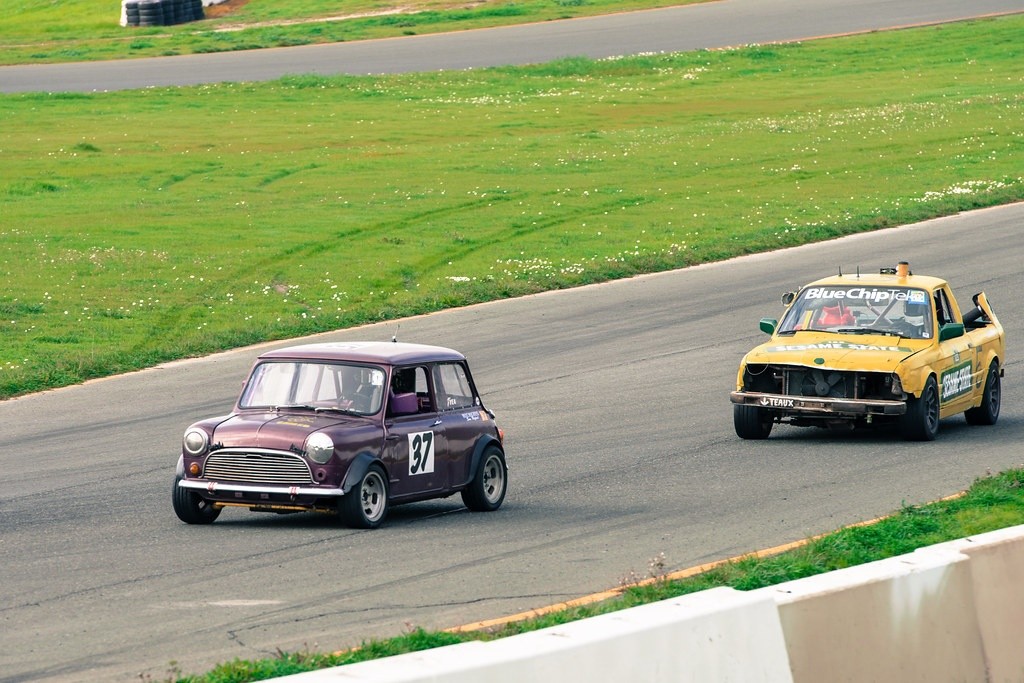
[900,290,938,326]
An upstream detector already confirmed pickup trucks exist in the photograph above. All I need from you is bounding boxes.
[730,262,1008,441]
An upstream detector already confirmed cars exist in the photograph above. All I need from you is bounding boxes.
[172,341,509,529]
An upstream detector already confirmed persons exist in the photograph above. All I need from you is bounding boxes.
[894,295,942,338]
[340,371,401,413]
